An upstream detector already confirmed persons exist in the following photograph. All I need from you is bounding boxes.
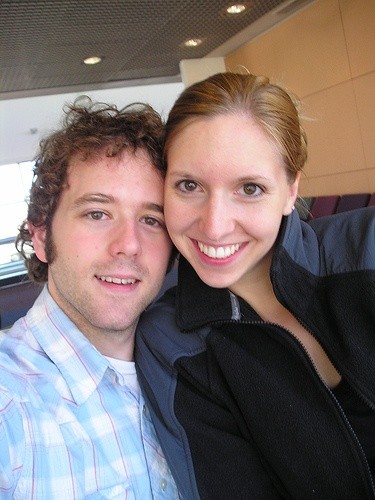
[0,95,187,500]
[133,70,375,500]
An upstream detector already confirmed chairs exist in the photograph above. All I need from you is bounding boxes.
[294,193,375,220]
